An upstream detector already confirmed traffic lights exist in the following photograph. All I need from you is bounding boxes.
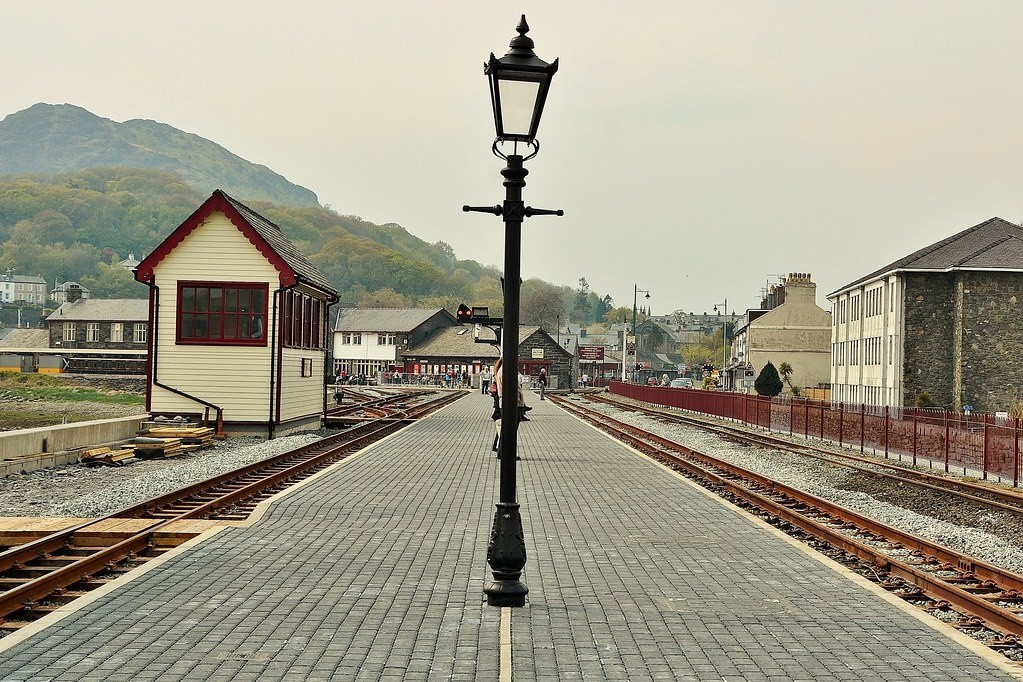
[457,303,472,319]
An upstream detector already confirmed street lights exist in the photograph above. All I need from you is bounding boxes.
[713,297,728,393]
[631,283,651,382]
[460,12,566,607]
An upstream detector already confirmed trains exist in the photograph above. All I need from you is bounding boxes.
[67,357,147,373]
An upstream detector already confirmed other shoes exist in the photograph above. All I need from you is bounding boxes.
[521,417,531,421]
[524,405,532,411]
[498,454,521,460]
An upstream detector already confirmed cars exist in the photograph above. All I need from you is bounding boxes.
[671,377,694,390]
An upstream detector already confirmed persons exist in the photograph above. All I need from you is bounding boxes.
[241,309,262,339]
[491,356,534,461]
[385,370,402,384]
[582,372,588,388]
[443,370,469,389]
[539,369,546,400]
[578,375,582,387]
[479,366,490,394]
[336,368,365,385]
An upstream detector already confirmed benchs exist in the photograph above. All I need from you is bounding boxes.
[362,377,377,384]
